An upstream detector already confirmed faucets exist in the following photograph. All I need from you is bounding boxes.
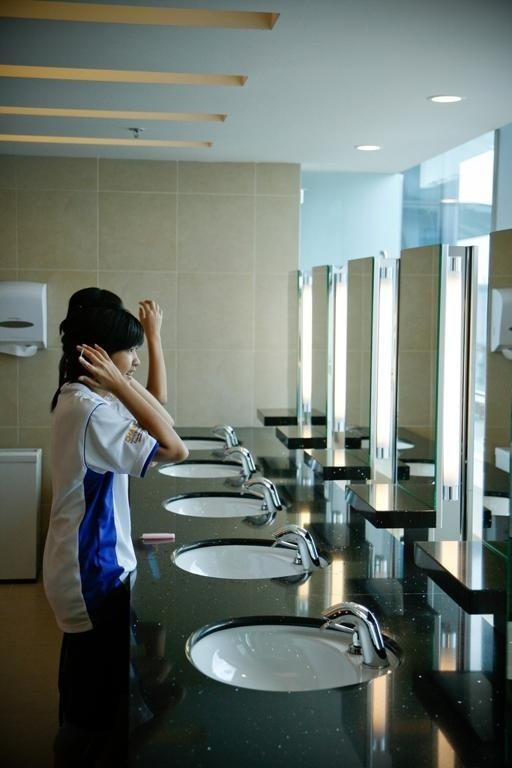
[322,601,390,669]
[211,424,322,570]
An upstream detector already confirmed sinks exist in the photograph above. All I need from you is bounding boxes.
[185,615,403,692]
[161,436,302,580]
[354,436,511,517]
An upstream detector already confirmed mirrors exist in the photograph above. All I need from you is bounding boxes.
[344,256,374,466]
[287,269,298,418]
[395,243,441,513]
[482,229,511,561]
[312,265,329,437]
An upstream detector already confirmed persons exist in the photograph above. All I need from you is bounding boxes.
[42,306,191,768]
[54,287,169,408]
